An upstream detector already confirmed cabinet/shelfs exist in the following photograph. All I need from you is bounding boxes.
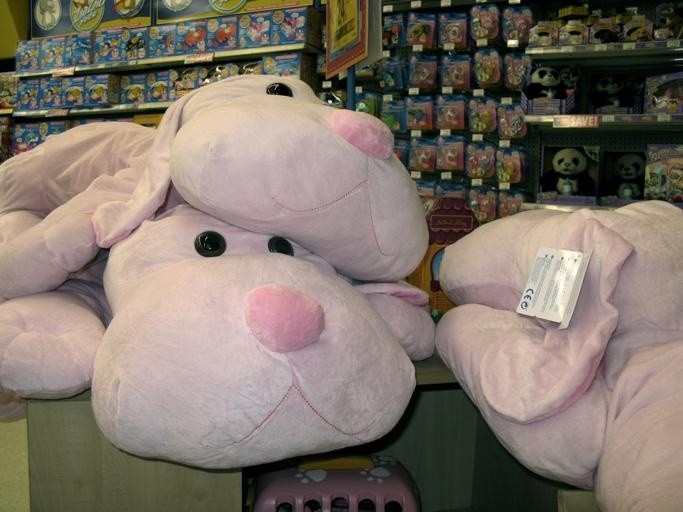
[10,3,322,155]
[519,1,683,210]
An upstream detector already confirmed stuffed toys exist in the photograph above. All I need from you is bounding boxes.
[0,176,435,466]
[439,201,682,511]
[523,65,645,199]
[0,77,429,292]
